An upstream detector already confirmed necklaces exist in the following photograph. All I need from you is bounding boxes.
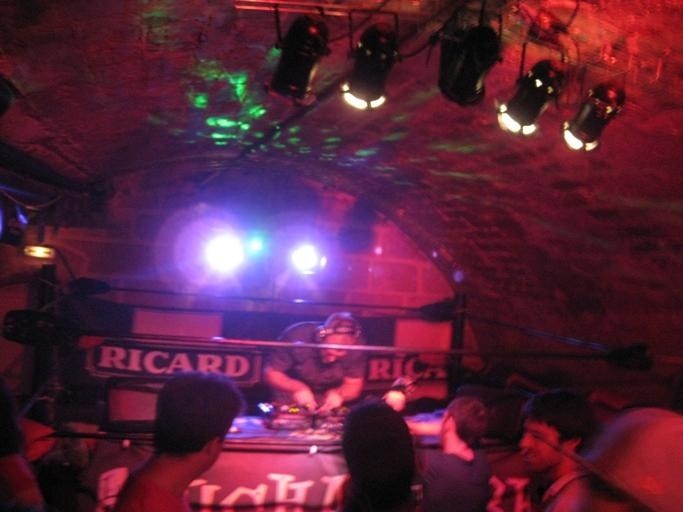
[336,401,420,511]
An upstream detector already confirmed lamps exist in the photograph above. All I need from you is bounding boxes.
[268,10,627,152]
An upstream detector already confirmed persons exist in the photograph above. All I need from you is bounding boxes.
[113,371,240,509]
[260,309,364,418]
[412,394,497,512]
[484,388,611,510]
[0,381,50,509]
